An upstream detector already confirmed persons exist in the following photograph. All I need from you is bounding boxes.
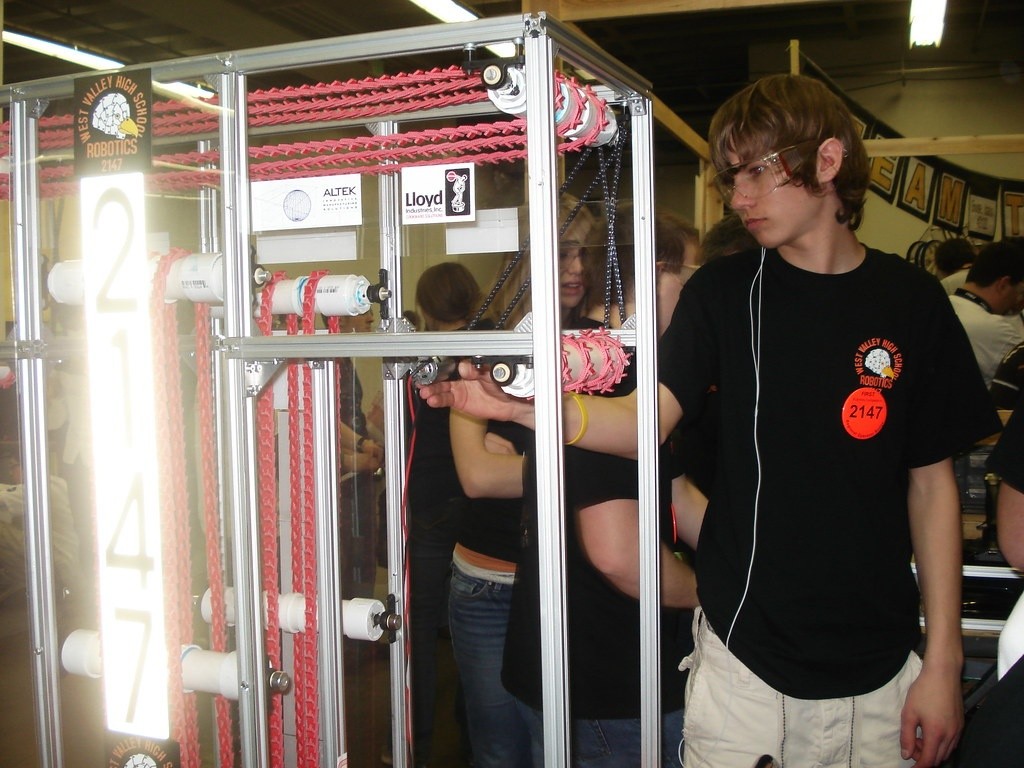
[446,193,614,767]
[935,238,1024,768]
[416,74,1006,768]
[498,200,761,767]
[282,308,386,676]
[381,261,503,768]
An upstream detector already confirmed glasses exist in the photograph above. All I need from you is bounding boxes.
[710,141,848,206]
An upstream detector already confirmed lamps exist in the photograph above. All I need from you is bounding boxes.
[908,0,947,50]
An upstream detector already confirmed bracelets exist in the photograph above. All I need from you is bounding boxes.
[563,391,588,446]
[356,437,367,453]
